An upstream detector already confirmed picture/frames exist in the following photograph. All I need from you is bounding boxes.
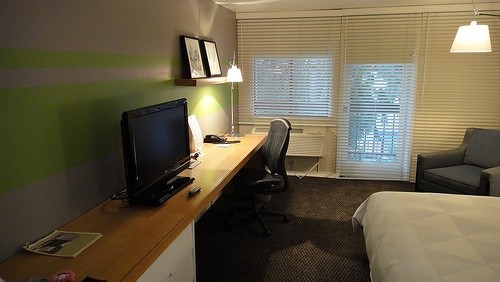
[179,35,208,79]
[200,39,223,77]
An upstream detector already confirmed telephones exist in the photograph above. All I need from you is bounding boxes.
[204,134,227,144]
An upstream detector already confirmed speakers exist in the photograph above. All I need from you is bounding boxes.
[120,98,194,207]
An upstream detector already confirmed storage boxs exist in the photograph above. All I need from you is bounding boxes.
[250,126,325,158]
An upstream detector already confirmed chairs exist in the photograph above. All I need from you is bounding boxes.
[221,117,292,237]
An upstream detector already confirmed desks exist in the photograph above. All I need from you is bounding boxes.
[0,133,268,282]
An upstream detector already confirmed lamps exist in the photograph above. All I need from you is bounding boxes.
[450,9,500,53]
[225,49,244,134]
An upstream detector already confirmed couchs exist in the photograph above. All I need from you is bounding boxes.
[414,127,500,197]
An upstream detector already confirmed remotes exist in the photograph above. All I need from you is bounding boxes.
[189,187,202,195]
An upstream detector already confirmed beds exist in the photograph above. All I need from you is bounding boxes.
[351,190,500,282]
